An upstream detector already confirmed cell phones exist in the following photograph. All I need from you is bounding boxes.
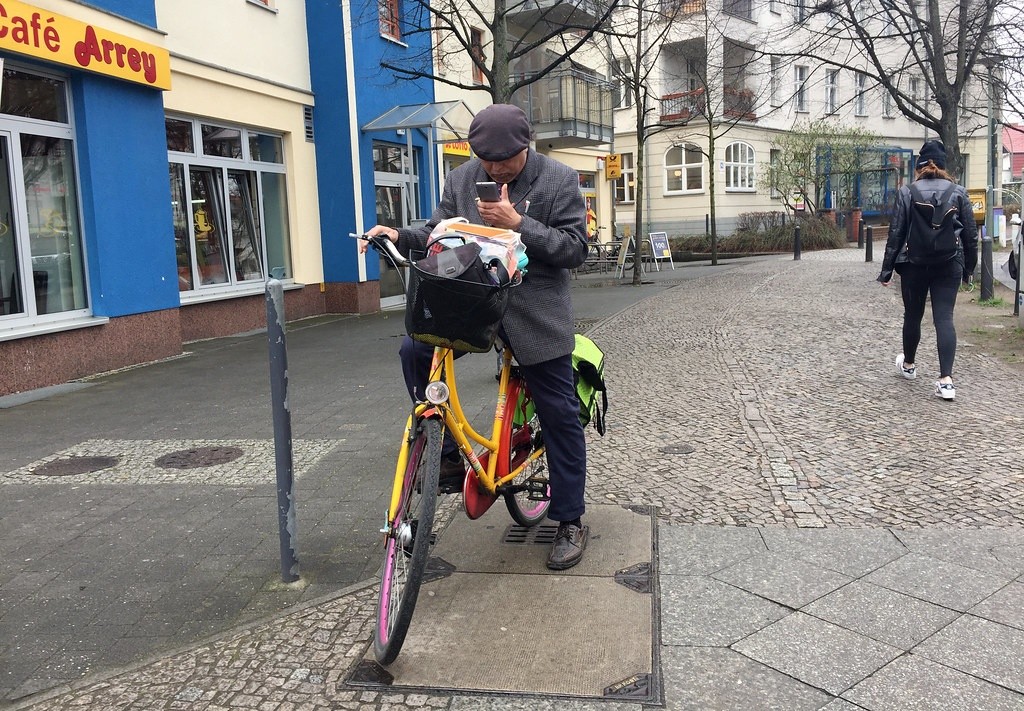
[476,181,500,204]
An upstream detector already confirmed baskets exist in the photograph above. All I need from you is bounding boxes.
[405,249,515,354]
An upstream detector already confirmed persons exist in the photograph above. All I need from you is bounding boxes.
[361,103,590,570]
[876,140,979,399]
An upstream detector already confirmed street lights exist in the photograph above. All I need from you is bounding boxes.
[27,181,41,235]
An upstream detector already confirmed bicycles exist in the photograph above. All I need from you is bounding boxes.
[584,224,636,270]
[345,229,553,665]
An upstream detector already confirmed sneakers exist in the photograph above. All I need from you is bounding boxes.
[546,522,588,569]
[415,454,466,491]
[935,380,956,399]
[895,353,916,380]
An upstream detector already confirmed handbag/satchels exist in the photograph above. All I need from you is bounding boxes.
[505,333,609,436]
[413,234,511,328]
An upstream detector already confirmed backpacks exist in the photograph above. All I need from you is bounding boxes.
[907,181,959,267]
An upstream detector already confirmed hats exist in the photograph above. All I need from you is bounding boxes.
[916,139,946,170]
[468,103,531,162]
